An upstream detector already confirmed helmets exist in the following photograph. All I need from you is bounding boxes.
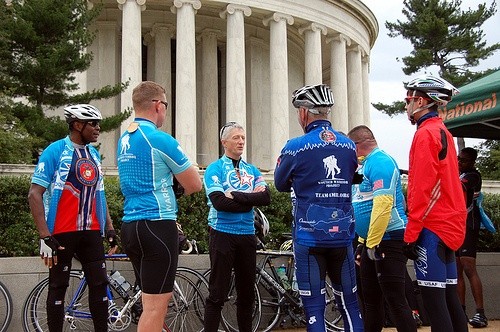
[256,208,269,238]
[280,239,293,252]
[63,104,102,124]
[292,84,334,108]
[403,74,460,106]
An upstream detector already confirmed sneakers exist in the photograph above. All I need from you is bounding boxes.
[469,311,488,328]
[412,311,423,326]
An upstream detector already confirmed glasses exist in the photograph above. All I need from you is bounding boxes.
[405,96,431,104]
[152,100,168,109]
[87,122,101,132]
[457,155,472,163]
[221,122,237,136]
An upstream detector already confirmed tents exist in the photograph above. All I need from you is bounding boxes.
[438,70,500,141]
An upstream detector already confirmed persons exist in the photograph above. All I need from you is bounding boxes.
[403,76,469,332]
[203,122,269,332]
[347,125,417,332]
[274,84,364,332]
[28,104,118,332]
[405,246,423,327]
[117,82,202,332]
[454,148,488,327]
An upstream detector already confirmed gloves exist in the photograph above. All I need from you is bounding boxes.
[403,240,419,261]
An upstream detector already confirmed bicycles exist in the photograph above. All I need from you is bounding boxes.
[0,281,14,332]
[20,229,361,332]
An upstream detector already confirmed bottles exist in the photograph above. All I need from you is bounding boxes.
[110,270,130,291]
[292,269,298,291]
[277,267,291,290]
